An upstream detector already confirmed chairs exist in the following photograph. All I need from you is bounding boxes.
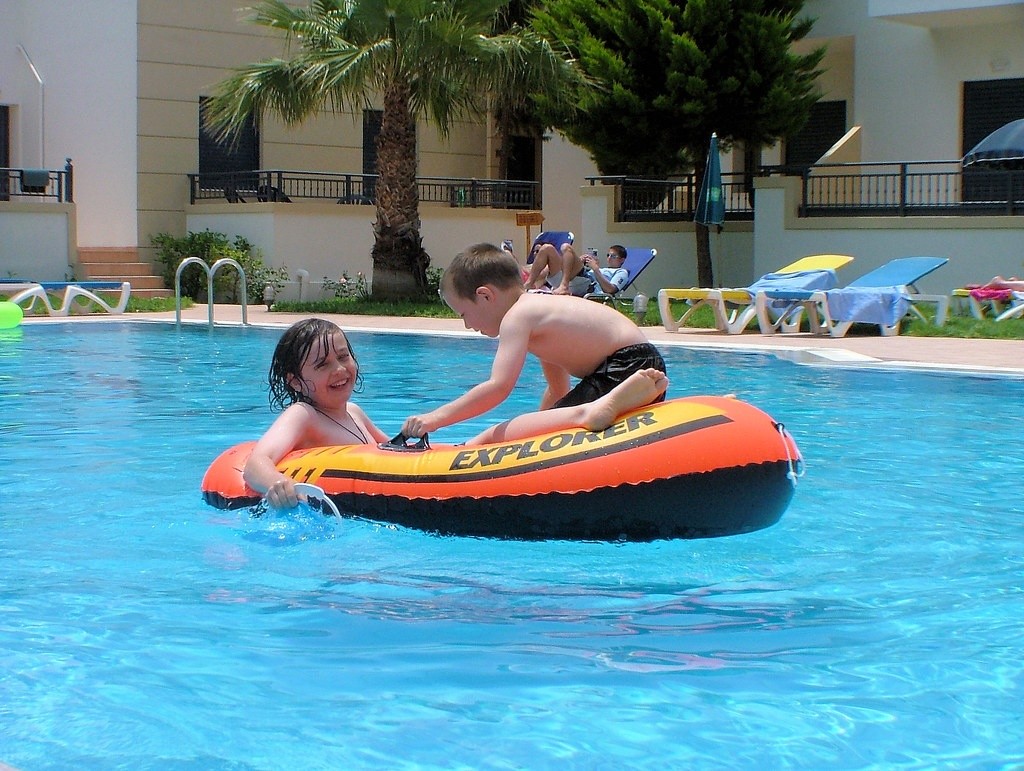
[527,247,656,316]
[223,188,247,203]
[257,186,291,202]
[952,287,1024,322]
[337,196,374,205]
[658,252,854,334]
[755,256,950,340]
[500,230,575,291]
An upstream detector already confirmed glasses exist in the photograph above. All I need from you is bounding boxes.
[607,254,622,259]
[532,249,539,253]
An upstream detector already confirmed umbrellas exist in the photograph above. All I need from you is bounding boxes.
[961,119,1024,167]
[693,131,726,288]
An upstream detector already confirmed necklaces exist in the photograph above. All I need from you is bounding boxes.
[316,408,368,444]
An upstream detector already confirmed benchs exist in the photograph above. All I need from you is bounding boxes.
[0,278,130,317]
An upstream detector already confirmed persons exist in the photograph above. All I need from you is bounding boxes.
[242,319,669,511]
[981,275,1024,292]
[402,244,666,436]
[504,242,556,289]
[522,243,629,295]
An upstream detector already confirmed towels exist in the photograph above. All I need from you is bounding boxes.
[827,284,913,331]
[19,166,50,193]
[686,268,840,308]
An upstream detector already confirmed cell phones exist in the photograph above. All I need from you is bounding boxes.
[591,249,599,259]
